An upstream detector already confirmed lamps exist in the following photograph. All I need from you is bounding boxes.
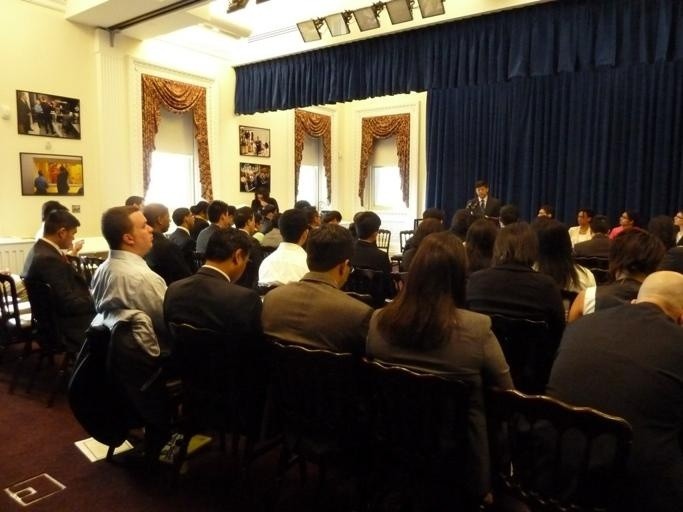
[293,1,445,50]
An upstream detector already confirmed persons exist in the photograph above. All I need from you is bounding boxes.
[57,163,69,193]
[23,180,682,512]
[34,170,49,193]
[254,136,262,155]
[17,93,56,134]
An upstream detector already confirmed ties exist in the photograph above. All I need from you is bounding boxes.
[481,199,485,208]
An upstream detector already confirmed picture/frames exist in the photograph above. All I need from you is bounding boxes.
[236,123,273,158]
[237,161,272,195]
[16,151,87,198]
[15,89,82,141]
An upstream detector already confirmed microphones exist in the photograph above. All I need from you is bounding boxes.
[468,200,473,208]
[474,200,480,207]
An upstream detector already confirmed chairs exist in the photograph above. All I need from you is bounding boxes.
[0,206,682,503]
